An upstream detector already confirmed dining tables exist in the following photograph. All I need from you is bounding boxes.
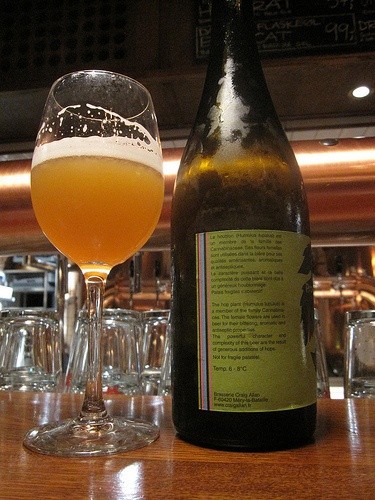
[0,389,375,500]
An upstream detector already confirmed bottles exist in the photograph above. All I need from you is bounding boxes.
[170,0,317,454]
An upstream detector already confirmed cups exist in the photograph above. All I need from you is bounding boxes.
[28,68,161,453]
[315,308,331,398]
[344,310,375,399]
[0,307,173,396]
